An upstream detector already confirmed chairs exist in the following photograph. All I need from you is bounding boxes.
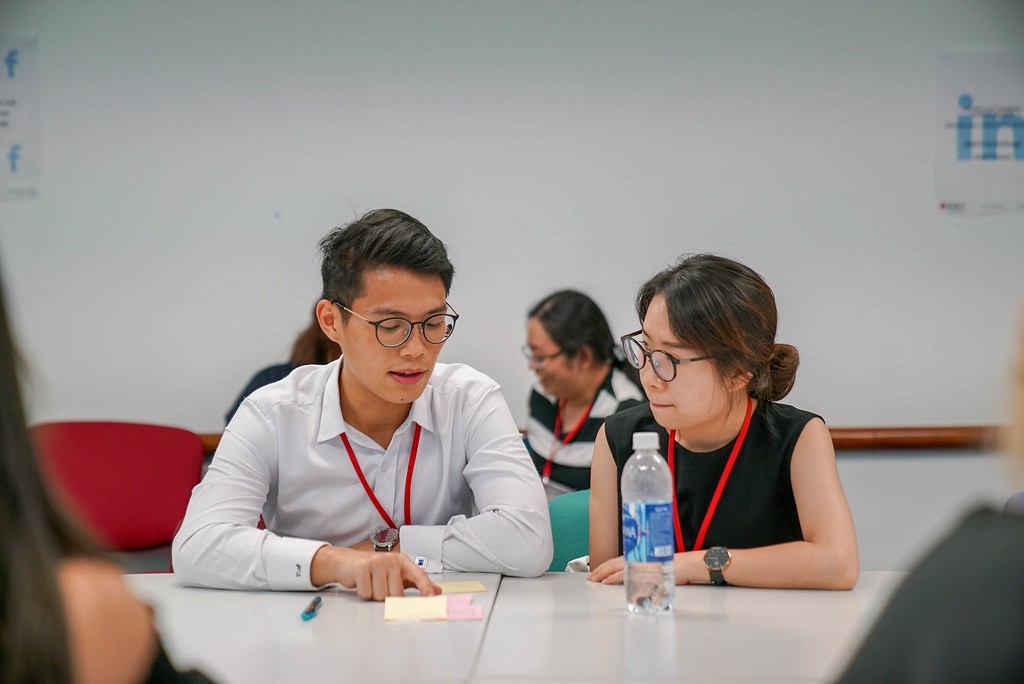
[29,418,206,552]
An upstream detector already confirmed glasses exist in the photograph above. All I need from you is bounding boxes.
[521,345,564,370]
[334,301,459,348]
[621,330,713,382]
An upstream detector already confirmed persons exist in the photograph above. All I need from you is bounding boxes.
[831,313,1024,684]
[172,208,554,601]
[220,317,343,423]
[522,291,650,498]
[587,255,860,590]
[0,268,213,683]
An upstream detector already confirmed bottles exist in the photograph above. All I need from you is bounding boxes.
[621,432,674,617]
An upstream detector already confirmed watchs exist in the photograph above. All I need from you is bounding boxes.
[703,546,731,585]
[372,528,399,551]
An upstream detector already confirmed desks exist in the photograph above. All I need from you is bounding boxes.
[128,568,889,684]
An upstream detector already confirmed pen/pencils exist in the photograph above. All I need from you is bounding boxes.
[300,596,322,620]
